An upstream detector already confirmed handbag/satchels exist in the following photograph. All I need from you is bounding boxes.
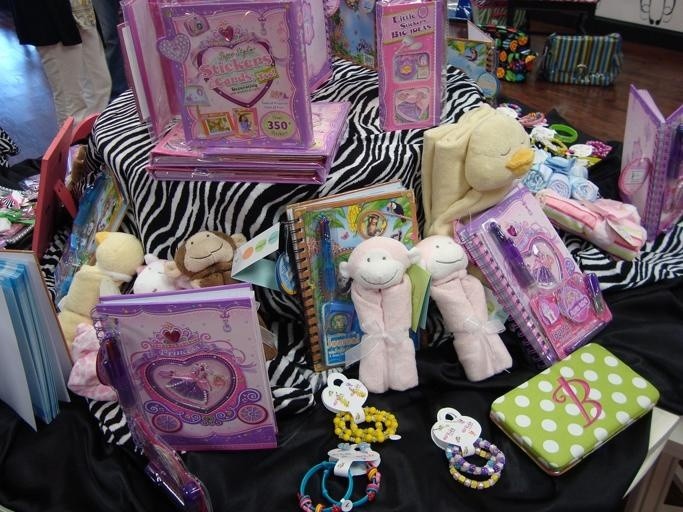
[540,33,625,85]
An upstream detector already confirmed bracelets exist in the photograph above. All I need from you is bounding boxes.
[501,101,613,161]
[299,457,380,512]
[447,438,503,489]
[333,408,398,443]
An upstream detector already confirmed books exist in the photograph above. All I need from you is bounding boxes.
[116,1,350,185]
[614,85,682,241]
[375,0,444,134]
[90,282,279,510]
[458,185,612,369]
[283,177,421,371]
[0,250,73,436]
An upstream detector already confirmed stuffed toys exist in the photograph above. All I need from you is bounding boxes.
[338,233,421,394]
[55,230,251,399]
[411,235,512,381]
[414,104,535,238]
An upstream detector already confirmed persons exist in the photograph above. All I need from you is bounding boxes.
[7,0,112,130]
